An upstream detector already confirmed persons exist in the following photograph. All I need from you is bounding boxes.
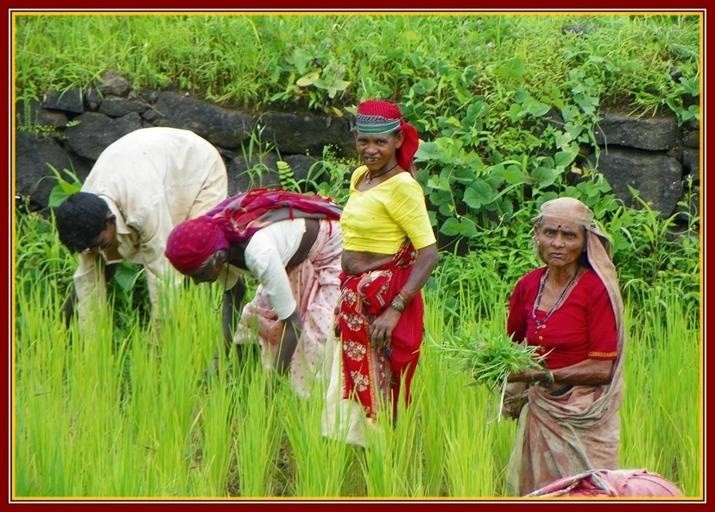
[500,197,624,498]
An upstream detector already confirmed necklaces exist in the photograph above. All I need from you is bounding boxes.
[532,267,584,329]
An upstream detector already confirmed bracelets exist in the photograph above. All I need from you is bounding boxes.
[528,372,533,381]
[545,369,554,385]
